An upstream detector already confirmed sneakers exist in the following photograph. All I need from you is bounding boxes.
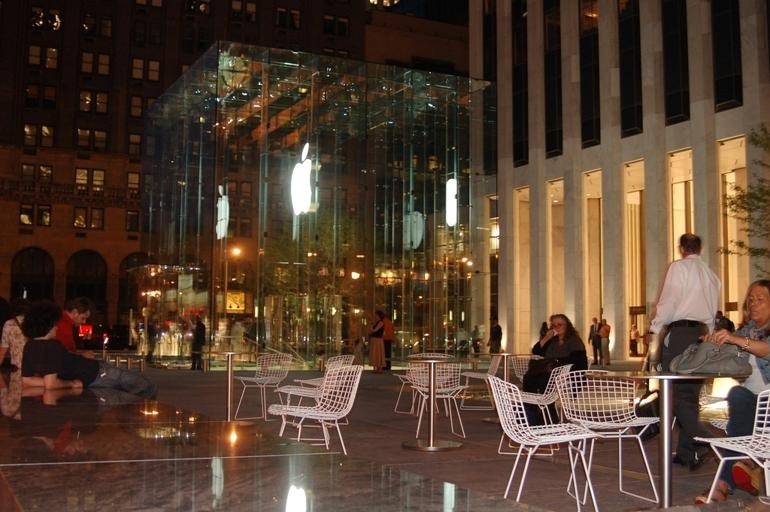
[673,434,716,472]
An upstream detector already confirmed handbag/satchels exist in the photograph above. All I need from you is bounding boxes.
[668,341,753,375]
[631,396,660,440]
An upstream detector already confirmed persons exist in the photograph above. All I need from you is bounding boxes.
[470,325,482,358]
[630,324,640,357]
[540,322,548,341]
[148,323,156,364]
[594,319,611,369]
[522,314,597,427]
[192,317,206,371]
[588,316,604,366]
[2,299,25,367]
[696,279,770,504]
[646,233,722,471]
[368,310,393,372]
[54,299,98,359]
[487,316,502,360]
[21,300,160,396]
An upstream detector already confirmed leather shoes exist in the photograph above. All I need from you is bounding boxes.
[694,488,727,503]
[732,461,760,496]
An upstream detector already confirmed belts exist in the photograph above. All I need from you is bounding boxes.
[669,320,701,329]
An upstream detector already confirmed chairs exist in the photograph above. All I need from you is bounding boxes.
[709,417,729,435]
[497,363,575,456]
[693,386,770,507]
[485,373,605,512]
[554,369,660,506]
[265,363,367,456]
[292,355,357,425]
[460,353,501,413]
[509,355,545,382]
[232,353,294,421]
[388,353,457,418]
[273,360,343,425]
[407,361,470,440]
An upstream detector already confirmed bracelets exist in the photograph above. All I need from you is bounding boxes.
[743,335,753,352]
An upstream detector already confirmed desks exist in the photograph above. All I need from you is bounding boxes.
[384,356,492,452]
[191,351,218,375]
[583,370,748,511]
[208,352,257,426]
